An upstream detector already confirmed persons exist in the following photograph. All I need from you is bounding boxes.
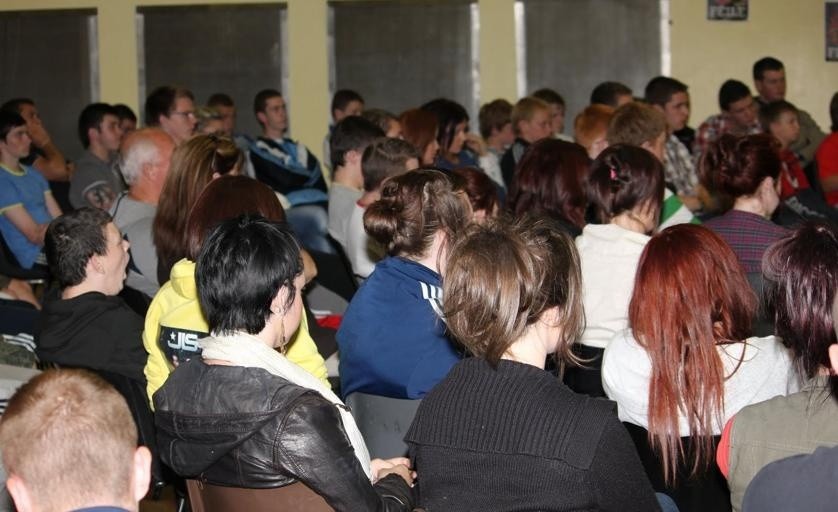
[1,57,837,491]
[598,224,807,512]
[152,211,418,512]
[714,224,837,512]
[0,367,152,512]
[401,209,681,512]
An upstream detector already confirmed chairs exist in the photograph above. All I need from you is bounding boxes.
[65,228,603,511]
